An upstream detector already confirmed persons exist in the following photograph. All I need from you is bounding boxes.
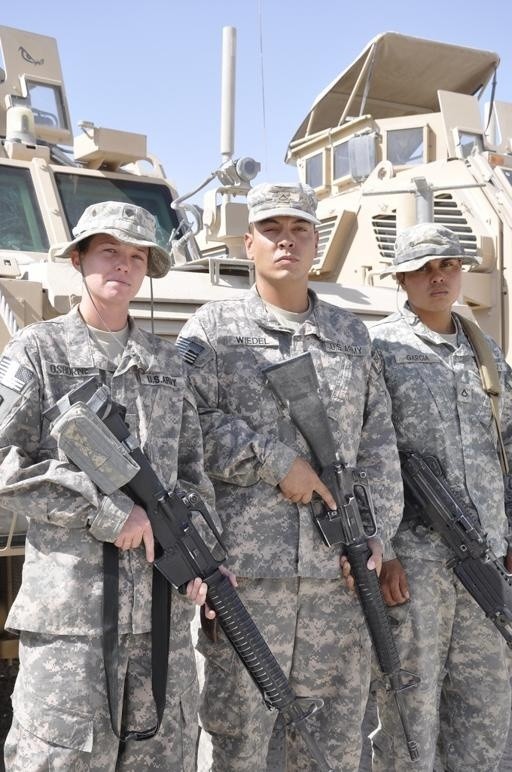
[368,222,512,772]
[0,200,239,772]
[172,182,406,772]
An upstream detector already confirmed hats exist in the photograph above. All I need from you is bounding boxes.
[246,182,323,226]
[48,201,171,279]
[381,223,480,279]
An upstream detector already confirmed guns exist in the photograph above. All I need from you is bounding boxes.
[398,450,511,647]
[261,353,422,762]
[42,377,331,772]
